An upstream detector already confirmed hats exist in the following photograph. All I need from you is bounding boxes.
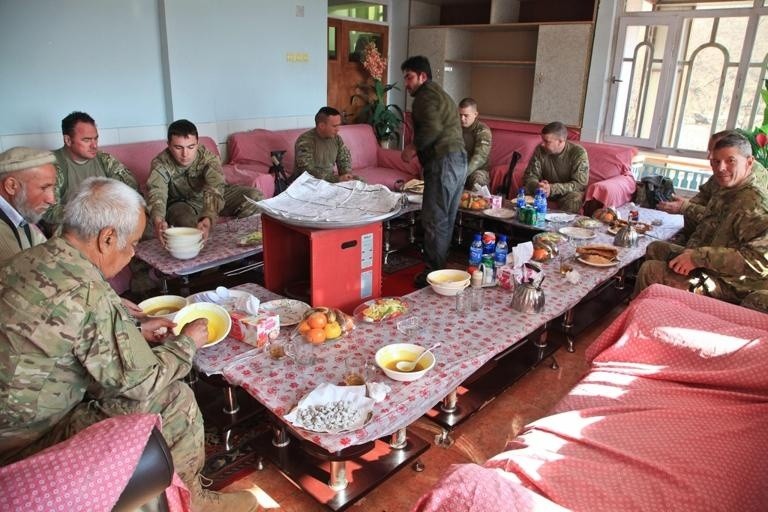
[0,146,57,173]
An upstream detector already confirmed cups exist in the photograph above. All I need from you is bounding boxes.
[345,356,375,385]
[470,288,484,311]
[401,196,408,209]
[214,216,259,238]
[262,329,291,361]
[456,289,471,317]
[284,334,314,367]
[560,255,574,274]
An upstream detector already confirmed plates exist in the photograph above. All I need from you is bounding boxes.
[288,402,374,435]
[259,299,312,327]
[353,297,415,325]
[608,226,645,237]
[532,213,604,246]
[575,251,620,267]
[407,195,424,204]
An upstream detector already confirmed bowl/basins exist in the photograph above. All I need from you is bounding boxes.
[161,226,204,260]
[426,278,471,297]
[137,295,188,322]
[171,302,233,349]
[427,268,472,286]
[374,342,436,382]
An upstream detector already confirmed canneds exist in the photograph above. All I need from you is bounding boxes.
[518,205,525,222]
[527,208,536,225]
[628,210,639,222]
[482,254,494,271]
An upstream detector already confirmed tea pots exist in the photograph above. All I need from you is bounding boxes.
[614,225,639,248]
[511,263,545,314]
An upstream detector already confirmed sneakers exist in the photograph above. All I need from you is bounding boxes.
[416,269,435,289]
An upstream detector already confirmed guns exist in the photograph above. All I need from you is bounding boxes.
[268,150,298,197]
[496,151,521,200]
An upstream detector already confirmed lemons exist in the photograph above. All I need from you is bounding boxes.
[325,321,342,339]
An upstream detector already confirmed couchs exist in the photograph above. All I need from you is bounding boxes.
[0,408,194,511]
[411,298,767,512]
[97,137,260,205]
[639,283,767,330]
[227,123,421,195]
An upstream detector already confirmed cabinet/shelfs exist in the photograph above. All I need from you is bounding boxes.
[262,214,383,320]
[406,1,597,128]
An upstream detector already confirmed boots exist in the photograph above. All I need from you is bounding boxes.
[186,474,258,511]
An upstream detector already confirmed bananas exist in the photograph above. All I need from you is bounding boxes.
[303,306,336,324]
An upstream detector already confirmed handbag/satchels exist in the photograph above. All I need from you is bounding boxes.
[636,176,675,208]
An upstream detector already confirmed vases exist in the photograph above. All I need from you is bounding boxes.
[377,132,391,147]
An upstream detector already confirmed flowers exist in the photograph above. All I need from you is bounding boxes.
[737,78,768,172]
[341,38,406,146]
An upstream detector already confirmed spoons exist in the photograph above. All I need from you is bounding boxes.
[193,286,232,307]
[154,310,170,316]
[396,342,441,372]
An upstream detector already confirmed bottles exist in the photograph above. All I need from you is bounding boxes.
[468,188,547,287]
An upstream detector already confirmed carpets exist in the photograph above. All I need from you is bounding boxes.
[190,379,274,491]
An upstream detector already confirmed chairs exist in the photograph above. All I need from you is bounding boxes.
[488,128,638,216]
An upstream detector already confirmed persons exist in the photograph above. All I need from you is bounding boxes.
[293,105,364,184]
[37,112,162,294]
[637,135,768,306]
[145,118,265,248]
[521,120,590,214]
[658,131,759,245]
[0,175,257,512]
[742,289,768,314]
[0,146,58,263]
[686,220,688,222]
[399,54,468,289]
[457,96,493,190]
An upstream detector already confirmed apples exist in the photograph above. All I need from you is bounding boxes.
[463,199,471,208]
[473,197,480,201]
